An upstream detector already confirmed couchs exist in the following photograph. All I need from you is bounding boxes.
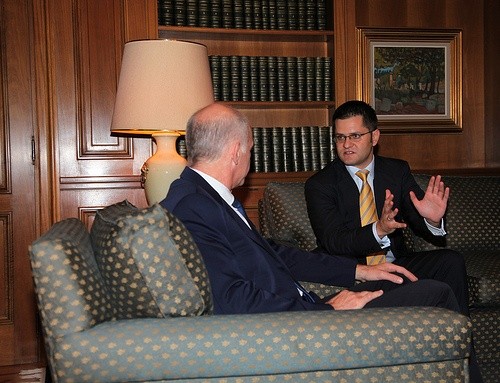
[258,174,500,383]
[25,197,472,383]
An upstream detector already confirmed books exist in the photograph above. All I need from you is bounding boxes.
[158,0,326,31]
[209,55,332,102]
[250,126,336,173]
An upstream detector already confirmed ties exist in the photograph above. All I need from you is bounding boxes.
[355,170,386,266]
[231,197,315,303]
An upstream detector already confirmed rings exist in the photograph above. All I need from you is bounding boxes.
[387,215,393,221]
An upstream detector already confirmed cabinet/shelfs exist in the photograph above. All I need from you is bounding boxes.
[154,0,338,205]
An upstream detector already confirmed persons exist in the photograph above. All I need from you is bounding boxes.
[159,102,463,383]
[305,100,482,383]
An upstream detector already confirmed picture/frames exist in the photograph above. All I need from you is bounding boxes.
[355,26,464,135]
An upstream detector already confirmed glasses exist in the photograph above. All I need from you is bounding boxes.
[332,128,377,143]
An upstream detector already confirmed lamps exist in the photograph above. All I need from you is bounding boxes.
[110,37,217,212]
